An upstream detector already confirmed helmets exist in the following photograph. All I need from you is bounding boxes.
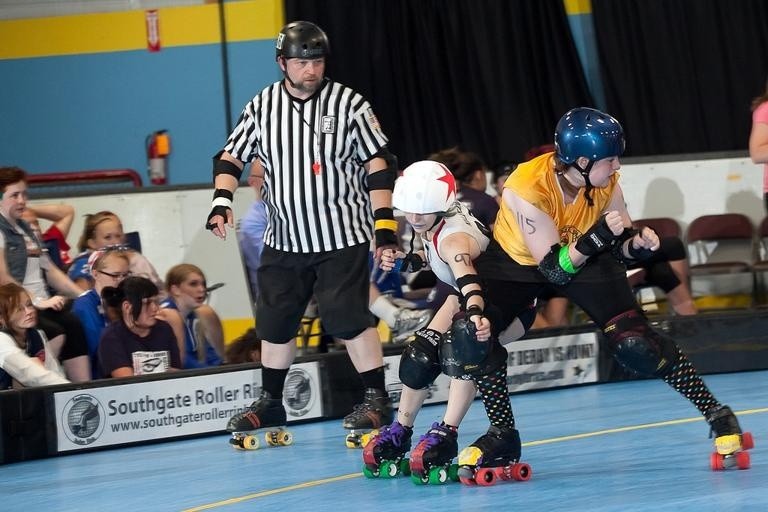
[555,107,627,165]
[276,21,329,62]
[391,159,457,214]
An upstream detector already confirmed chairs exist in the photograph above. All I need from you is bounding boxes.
[629,216,693,317]
[688,213,756,314]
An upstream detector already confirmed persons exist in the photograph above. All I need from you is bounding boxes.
[748,88,767,217]
[368,152,531,460]
[204,20,397,429]
[368,148,697,330]
[238,157,432,343]
[450,108,743,466]
[0,167,91,391]
[68,211,227,380]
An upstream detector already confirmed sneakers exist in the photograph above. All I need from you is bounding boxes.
[390,307,434,345]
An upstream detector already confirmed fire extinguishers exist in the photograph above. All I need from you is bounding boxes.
[145,129,169,185]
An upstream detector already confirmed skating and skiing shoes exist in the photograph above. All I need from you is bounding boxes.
[458,425,532,487]
[226,391,293,451]
[363,420,414,479]
[705,405,755,471]
[343,386,394,448]
[409,422,459,486]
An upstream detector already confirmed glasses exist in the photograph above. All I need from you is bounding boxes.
[97,269,134,282]
